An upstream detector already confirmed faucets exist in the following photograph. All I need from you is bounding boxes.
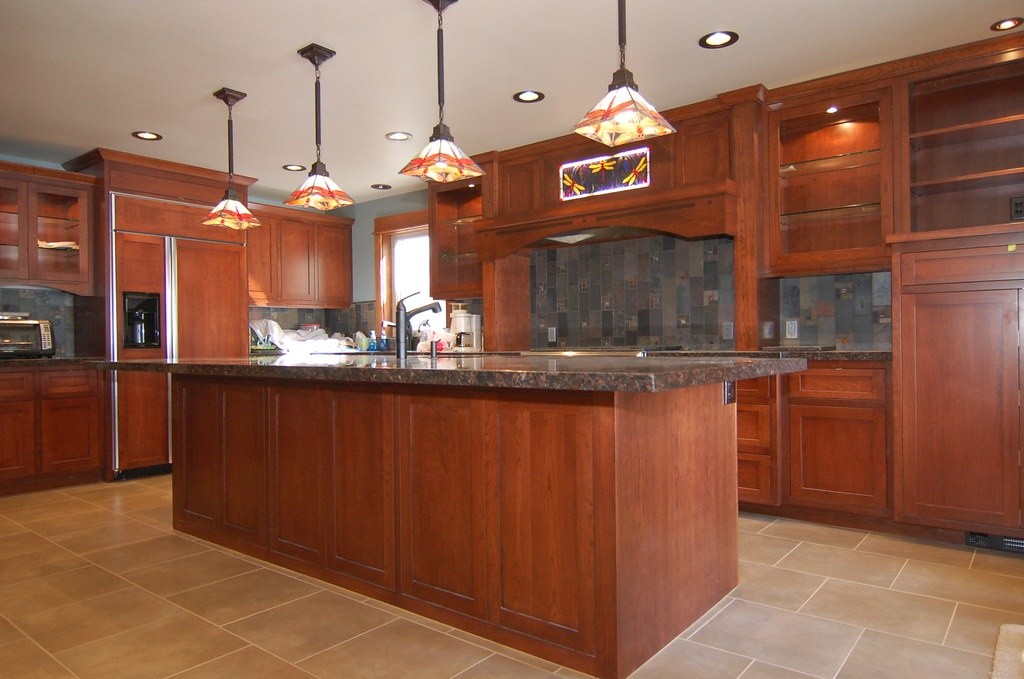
[381,320,397,329]
[395,290,443,360]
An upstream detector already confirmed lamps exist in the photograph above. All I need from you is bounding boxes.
[573,0,678,146]
[282,43,355,213]
[195,87,263,234]
[399,0,487,183]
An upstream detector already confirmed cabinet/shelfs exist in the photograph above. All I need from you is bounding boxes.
[0,145,345,497]
[736,362,894,520]
[896,49,1024,240]
[892,240,1024,539]
[426,148,483,300]
[766,83,896,273]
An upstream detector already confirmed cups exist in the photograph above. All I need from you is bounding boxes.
[300,323,320,333]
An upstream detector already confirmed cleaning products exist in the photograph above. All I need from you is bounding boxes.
[368,329,379,351]
[379,329,390,351]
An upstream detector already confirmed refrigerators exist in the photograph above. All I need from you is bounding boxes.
[114,231,250,473]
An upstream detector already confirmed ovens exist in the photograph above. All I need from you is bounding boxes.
[0,320,56,359]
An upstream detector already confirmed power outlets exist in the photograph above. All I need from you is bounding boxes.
[785,321,797,339]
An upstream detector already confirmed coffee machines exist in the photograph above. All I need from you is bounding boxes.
[449,303,481,354]
